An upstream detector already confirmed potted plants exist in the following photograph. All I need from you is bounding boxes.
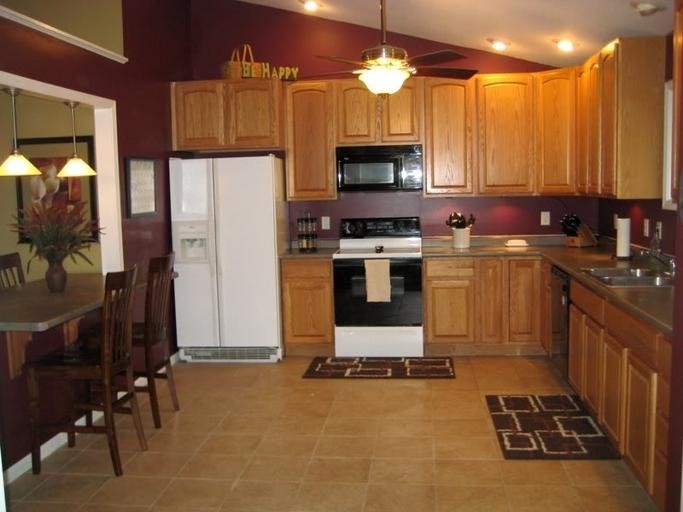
[10,189,107,292]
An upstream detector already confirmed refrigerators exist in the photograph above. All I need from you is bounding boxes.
[166,154,289,362]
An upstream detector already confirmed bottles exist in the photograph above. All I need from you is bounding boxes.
[294,218,318,252]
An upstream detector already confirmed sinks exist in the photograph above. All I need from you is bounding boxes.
[582,268,659,276]
[598,277,672,288]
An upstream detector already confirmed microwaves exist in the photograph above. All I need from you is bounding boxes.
[334,142,423,193]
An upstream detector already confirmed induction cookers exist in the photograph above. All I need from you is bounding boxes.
[333,246,426,260]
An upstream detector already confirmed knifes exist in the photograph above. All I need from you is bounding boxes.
[558,213,581,238]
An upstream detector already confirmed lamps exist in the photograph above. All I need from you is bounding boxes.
[353,0,417,96]
[0,85,41,176]
[56,101,96,178]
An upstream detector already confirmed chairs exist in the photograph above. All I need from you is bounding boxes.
[25,263,148,477]
[83,251,180,427]
[0,252,26,295]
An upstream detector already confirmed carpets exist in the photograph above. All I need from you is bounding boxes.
[485,394,622,459]
[303,357,457,379]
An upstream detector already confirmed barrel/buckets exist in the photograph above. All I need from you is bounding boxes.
[451,228,471,248]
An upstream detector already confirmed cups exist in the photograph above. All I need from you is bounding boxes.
[451,227,471,249]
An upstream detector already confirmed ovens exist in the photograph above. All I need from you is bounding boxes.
[330,255,425,361]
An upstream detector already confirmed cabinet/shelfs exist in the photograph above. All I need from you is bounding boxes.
[282,79,338,202]
[422,256,475,357]
[472,256,543,356]
[576,35,666,202]
[420,76,478,198]
[172,78,282,152]
[280,258,333,357]
[569,276,604,428]
[601,299,658,497]
[475,67,577,199]
[650,332,671,512]
[337,77,422,148]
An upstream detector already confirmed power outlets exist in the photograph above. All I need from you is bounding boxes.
[540,211,550,226]
[321,216,331,230]
[655,221,662,241]
[643,217,649,237]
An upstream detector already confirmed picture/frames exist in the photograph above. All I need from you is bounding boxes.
[14,133,100,244]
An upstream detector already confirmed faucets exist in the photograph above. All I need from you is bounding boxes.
[641,251,675,276]
